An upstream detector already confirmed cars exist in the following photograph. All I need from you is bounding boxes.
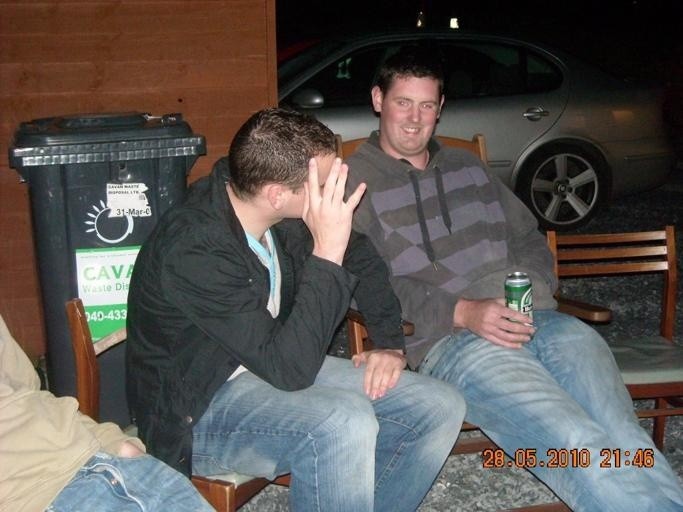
[276,31,358,90]
[276,26,675,232]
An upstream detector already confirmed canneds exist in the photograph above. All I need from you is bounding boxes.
[504,271,532,336]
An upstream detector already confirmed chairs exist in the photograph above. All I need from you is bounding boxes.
[537,221,683,452]
[59,292,416,512]
[331,125,571,512]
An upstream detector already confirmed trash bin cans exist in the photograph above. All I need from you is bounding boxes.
[8,109,207,430]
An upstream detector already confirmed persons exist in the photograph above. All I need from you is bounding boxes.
[119,103,468,512]
[334,49,683,510]
[0,308,219,512]
